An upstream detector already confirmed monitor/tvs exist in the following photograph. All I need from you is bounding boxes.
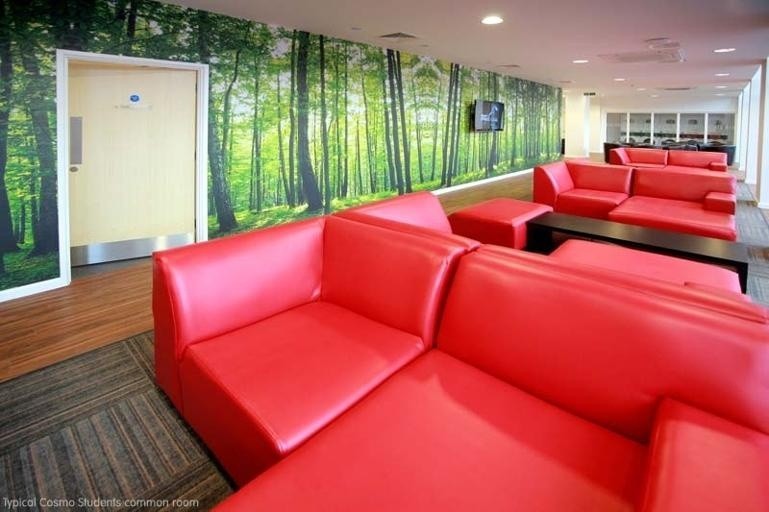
[472,99,505,132]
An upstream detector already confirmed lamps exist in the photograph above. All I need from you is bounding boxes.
[654,46,688,64]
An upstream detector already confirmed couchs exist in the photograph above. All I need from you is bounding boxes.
[609,147,669,169]
[533,160,636,220]
[152,213,467,490]
[473,237,768,323]
[668,150,728,171]
[604,142,631,163]
[207,253,769,512]
[608,167,737,239]
[331,190,479,251]
[626,138,704,151]
[704,145,736,166]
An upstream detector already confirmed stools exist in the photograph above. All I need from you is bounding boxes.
[448,196,553,250]
